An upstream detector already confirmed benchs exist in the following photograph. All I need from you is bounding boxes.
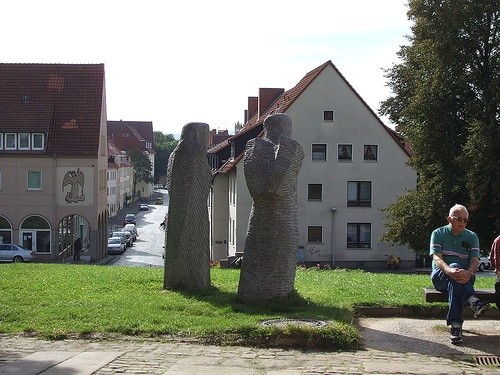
[423,288,496,302]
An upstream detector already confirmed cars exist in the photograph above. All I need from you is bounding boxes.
[123,213,137,226]
[156,197,163,204]
[107,224,138,255]
[478,251,493,272]
[139,204,149,211]
[0,244,36,263]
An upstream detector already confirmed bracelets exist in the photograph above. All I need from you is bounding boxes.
[469,270,473,277]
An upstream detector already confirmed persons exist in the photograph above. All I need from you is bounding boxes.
[74,238,82,260]
[430,204,490,345]
[490,236,500,310]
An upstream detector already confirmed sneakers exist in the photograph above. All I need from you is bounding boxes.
[450,327,462,344]
[472,298,490,318]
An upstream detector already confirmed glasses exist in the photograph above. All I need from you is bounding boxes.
[451,216,469,223]
